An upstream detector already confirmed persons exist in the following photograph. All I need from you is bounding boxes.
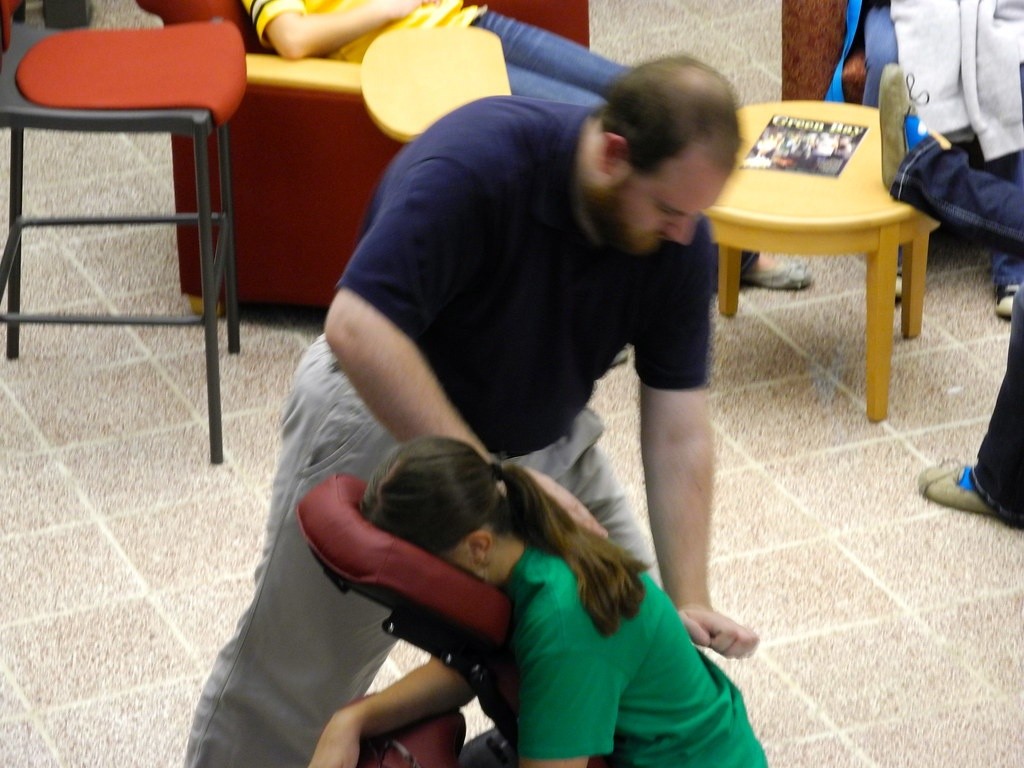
[240,1,816,302]
[861,58,1024,519]
[307,433,771,768]
[182,52,761,767]
[859,0,1024,316]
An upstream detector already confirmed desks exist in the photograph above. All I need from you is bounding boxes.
[704,99,949,424]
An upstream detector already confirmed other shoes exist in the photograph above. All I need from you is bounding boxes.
[895,266,903,298]
[918,460,999,516]
[878,64,930,190]
[995,284,1020,317]
[741,256,812,289]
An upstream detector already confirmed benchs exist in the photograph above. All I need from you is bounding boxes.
[782,0,869,103]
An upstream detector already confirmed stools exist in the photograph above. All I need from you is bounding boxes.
[1,1,248,466]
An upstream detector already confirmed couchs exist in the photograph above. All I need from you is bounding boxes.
[136,0,591,319]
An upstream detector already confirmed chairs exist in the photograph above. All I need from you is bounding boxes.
[294,469,607,767]
